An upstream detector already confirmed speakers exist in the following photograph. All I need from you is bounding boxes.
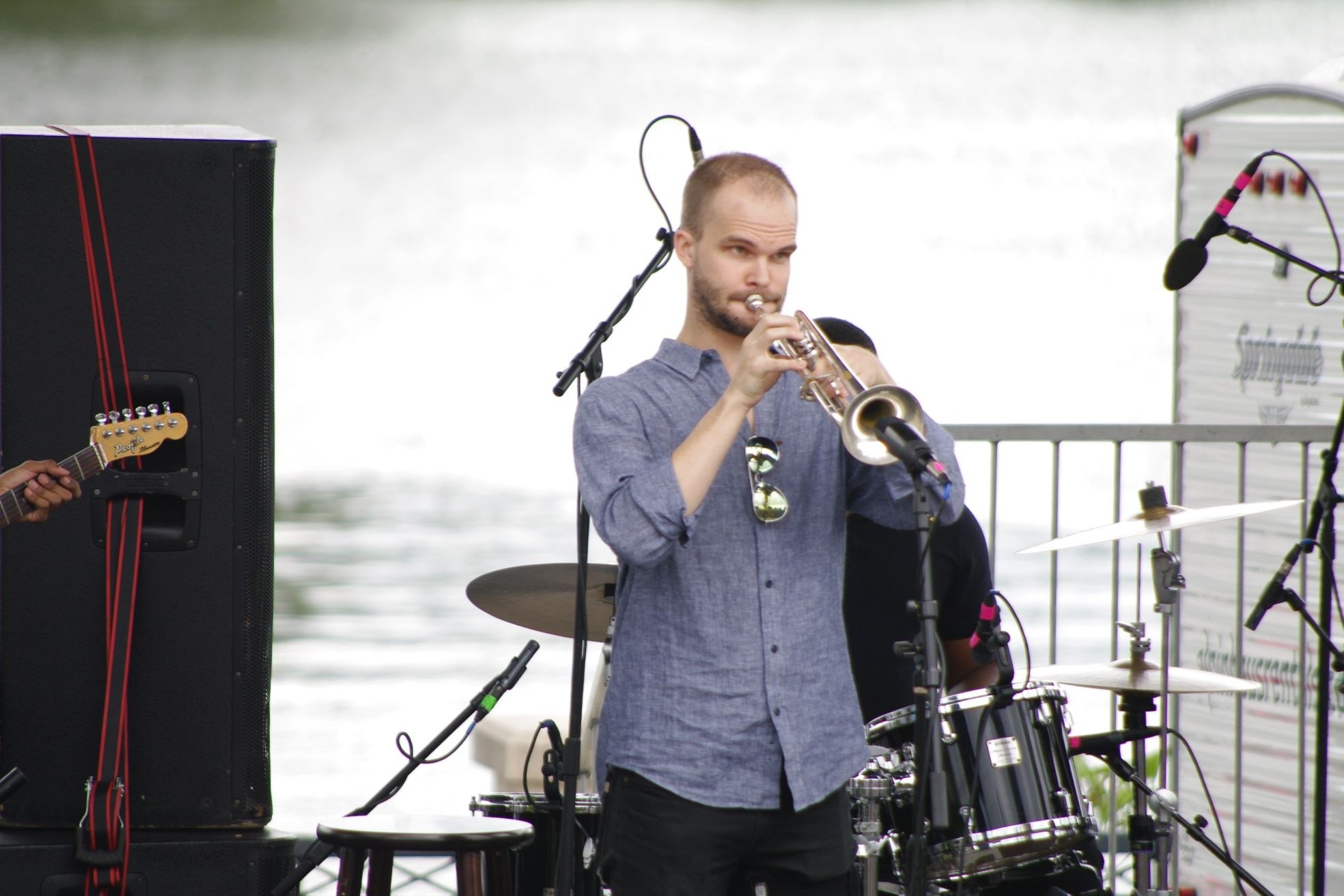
[0,827,299,896]
[0,135,277,829]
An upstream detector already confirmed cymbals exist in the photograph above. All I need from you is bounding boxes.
[1045,662,1261,699]
[1017,496,1306,555]
[468,564,620,644]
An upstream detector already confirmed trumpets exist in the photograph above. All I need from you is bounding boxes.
[745,294,929,466]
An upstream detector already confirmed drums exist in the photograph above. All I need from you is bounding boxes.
[469,791,604,896]
[865,678,1099,884]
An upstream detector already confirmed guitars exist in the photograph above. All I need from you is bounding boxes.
[1,401,193,526]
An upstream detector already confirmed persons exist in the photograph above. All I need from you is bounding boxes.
[569,154,966,896]
[804,317,1014,758]
[0,459,82,525]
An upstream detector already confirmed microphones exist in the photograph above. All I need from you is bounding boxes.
[689,126,704,166]
[545,718,565,781]
[1067,727,1163,755]
[1244,544,1304,631]
[871,416,954,487]
[475,639,540,723]
[969,593,999,664]
[1164,156,1263,291]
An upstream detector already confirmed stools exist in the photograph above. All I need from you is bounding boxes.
[316,816,538,896]
